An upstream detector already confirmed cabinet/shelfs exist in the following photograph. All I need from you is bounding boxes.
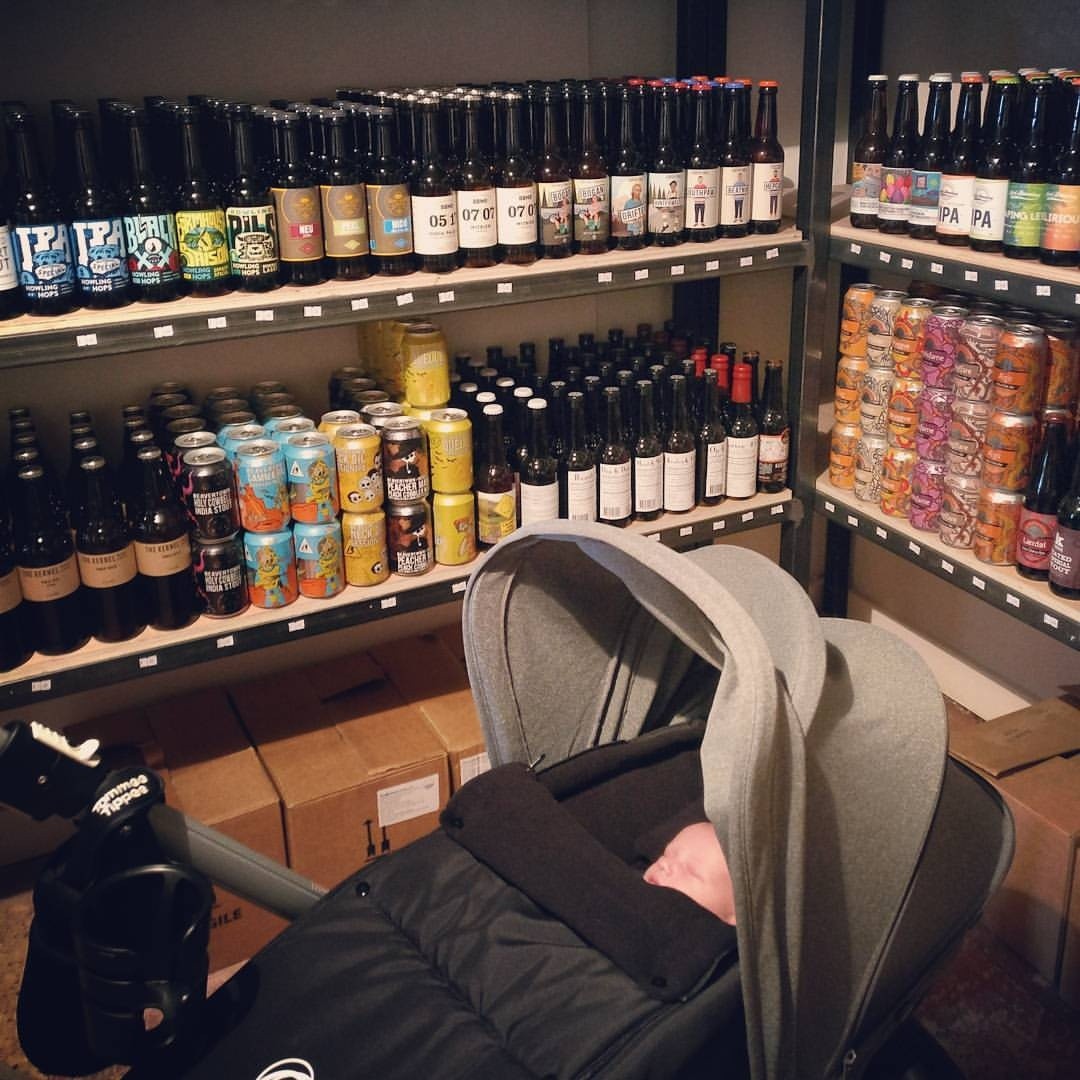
[1,1,1080,709]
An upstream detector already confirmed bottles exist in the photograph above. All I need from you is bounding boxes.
[848,64,1080,275]
[1010,404,1080,602]
[445,320,792,547]
[0,401,203,674]
[0,75,784,320]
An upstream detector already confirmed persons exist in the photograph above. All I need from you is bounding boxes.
[641,822,737,926]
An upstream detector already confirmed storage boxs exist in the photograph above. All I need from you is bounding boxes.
[947,682,1080,1009]
[62,684,289,971]
[225,650,452,892]
[370,616,493,793]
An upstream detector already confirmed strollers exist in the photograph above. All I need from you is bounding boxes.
[0,518,1020,1080]
